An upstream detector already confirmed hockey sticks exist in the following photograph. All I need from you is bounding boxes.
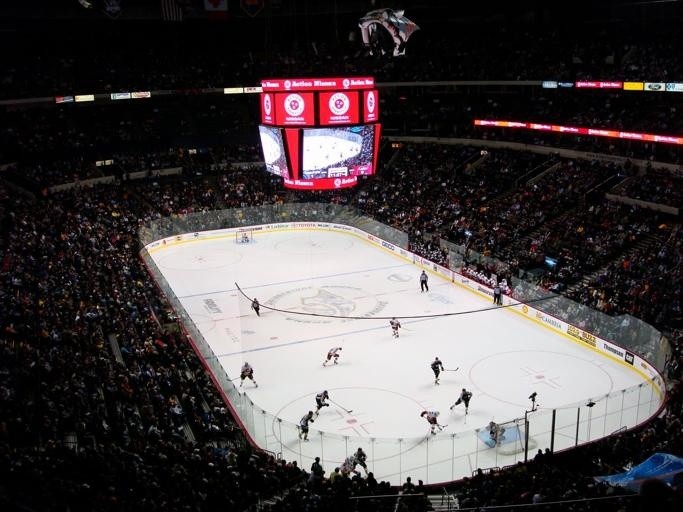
[423,417,448,428]
[225,377,240,381]
[328,399,353,413]
[443,368,459,371]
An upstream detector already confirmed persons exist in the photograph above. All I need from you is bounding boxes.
[475,267,680,449]
[420,409,443,435]
[297,410,314,440]
[352,447,369,475]
[449,388,472,414]
[0,266,242,450]
[240,361,256,387]
[314,390,329,417]
[250,298,260,317]
[1,1,682,266]
[389,316,401,337]
[0,450,682,510]
[322,347,342,366]
[419,270,428,292]
[430,357,444,384]
